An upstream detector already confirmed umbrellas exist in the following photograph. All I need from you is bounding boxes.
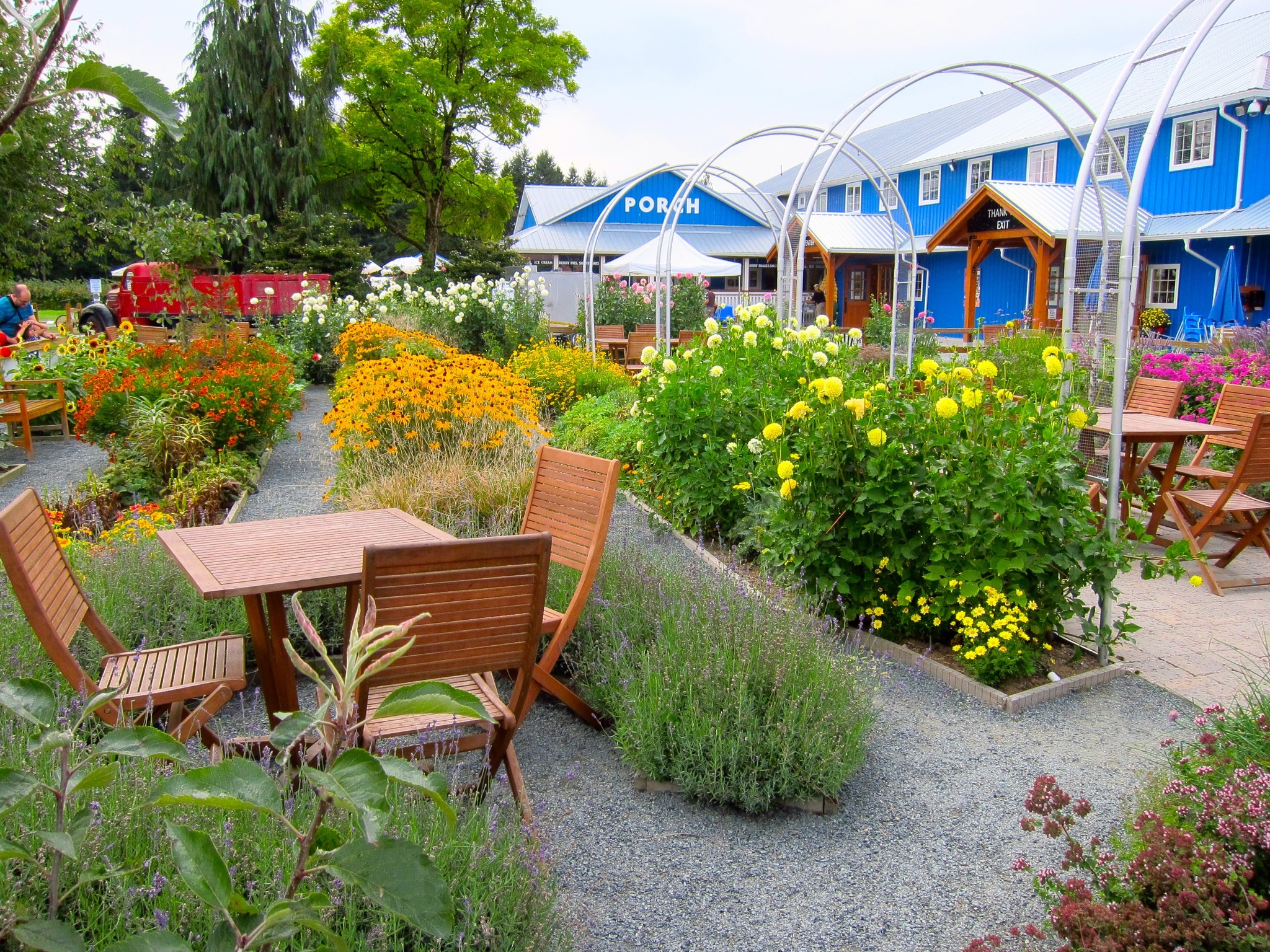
[1208,245,1248,344]
[361,251,455,277]
[1084,247,1112,334]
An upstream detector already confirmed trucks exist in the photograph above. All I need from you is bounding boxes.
[70,261,332,343]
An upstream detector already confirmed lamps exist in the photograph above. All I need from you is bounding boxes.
[949,159,956,171]
[1234,97,1270,116]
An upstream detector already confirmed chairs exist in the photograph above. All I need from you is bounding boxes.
[0,445,620,840]
[982,324,1009,353]
[1086,376,1270,596]
[1169,305,1223,359]
[0,366,70,460]
[108,321,250,349]
[593,325,726,381]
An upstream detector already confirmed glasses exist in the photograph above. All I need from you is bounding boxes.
[17,296,31,304]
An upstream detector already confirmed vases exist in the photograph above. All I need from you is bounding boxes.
[1156,325,1165,333]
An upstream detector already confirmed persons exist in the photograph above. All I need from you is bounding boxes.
[808,285,826,317]
[111,283,120,290]
[16,320,47,362]
[705,286,715,317]
[0,284,56,381]
[395,272,406,281]
[365,275,371,285]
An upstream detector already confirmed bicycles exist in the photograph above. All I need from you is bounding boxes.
[55,312,79,328]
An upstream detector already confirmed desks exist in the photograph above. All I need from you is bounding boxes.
[1075,414,1242,551]
[595,338,678,372]
[153,507,458,784]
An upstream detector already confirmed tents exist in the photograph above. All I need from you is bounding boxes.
[600,229,742,309]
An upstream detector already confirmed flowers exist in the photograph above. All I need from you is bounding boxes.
[1141,307,1172,332]
